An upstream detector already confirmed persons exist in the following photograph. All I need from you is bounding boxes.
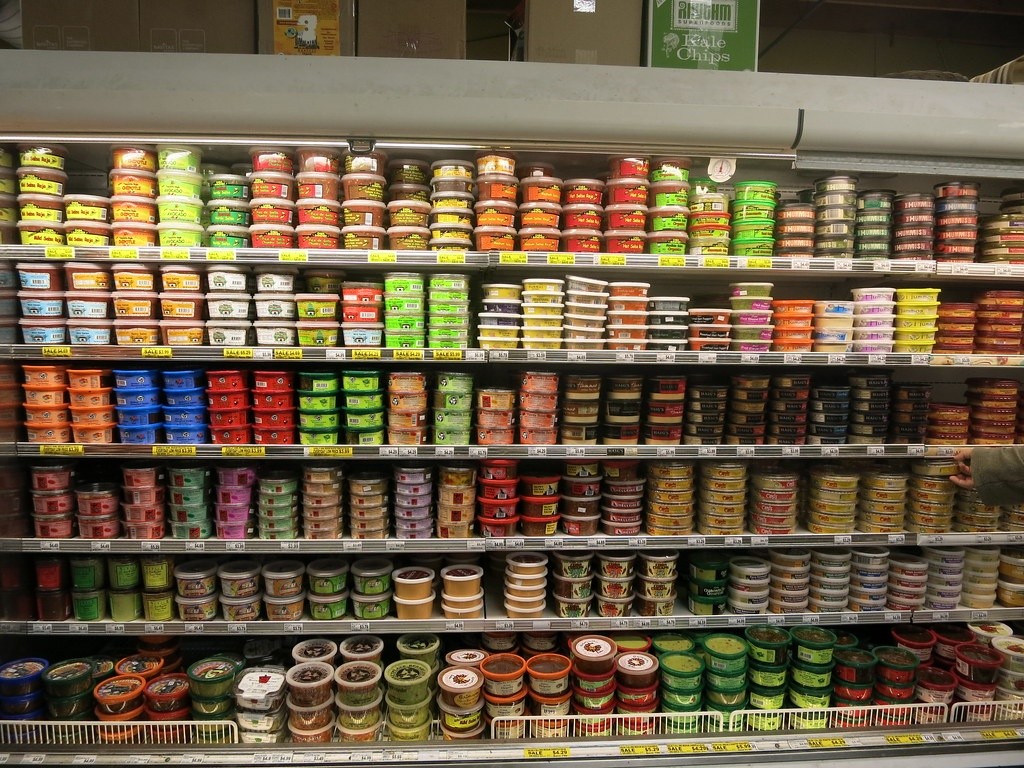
[949,446,1024,507]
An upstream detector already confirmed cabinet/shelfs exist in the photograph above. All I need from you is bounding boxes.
[0,47,1024,768]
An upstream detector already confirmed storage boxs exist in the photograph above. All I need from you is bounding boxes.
[524,0,642,67]
[21,0,470,59]
[644,0,760,71]
